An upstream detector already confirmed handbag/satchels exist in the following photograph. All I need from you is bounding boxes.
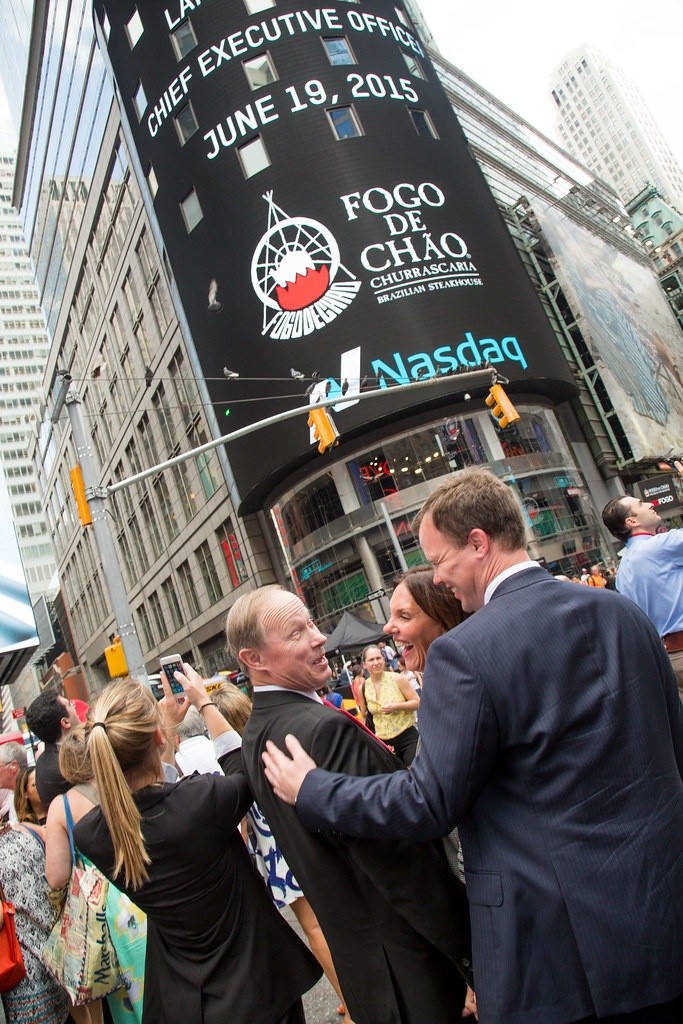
[41,793,126,1007]
[0,882,26,994]
[362,680,376,734]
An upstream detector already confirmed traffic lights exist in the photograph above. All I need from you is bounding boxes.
[485,385,520,429]
[307,408,339,454]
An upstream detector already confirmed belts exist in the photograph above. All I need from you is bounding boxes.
[660,630,683,653]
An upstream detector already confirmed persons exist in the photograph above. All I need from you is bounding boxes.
[224,585,480,1024]
[261,468,682,1024]
[0,644,423,1024]
[602,494,683,706]
[555,559,617,590]
[383,565,470,673]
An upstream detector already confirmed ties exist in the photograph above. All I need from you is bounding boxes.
[319,695,395,756]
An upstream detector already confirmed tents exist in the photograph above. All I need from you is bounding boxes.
[322,609,399,714]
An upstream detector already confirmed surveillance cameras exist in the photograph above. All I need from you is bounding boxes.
[464,394,471,403]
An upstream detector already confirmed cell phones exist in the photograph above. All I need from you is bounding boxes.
[159,655,189,699]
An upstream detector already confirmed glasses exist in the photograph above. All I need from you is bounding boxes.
[61,699,77,719]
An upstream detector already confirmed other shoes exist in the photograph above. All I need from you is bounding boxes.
[337,1002,346,1014]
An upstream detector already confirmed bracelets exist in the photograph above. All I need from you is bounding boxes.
[198,703,217,713]
